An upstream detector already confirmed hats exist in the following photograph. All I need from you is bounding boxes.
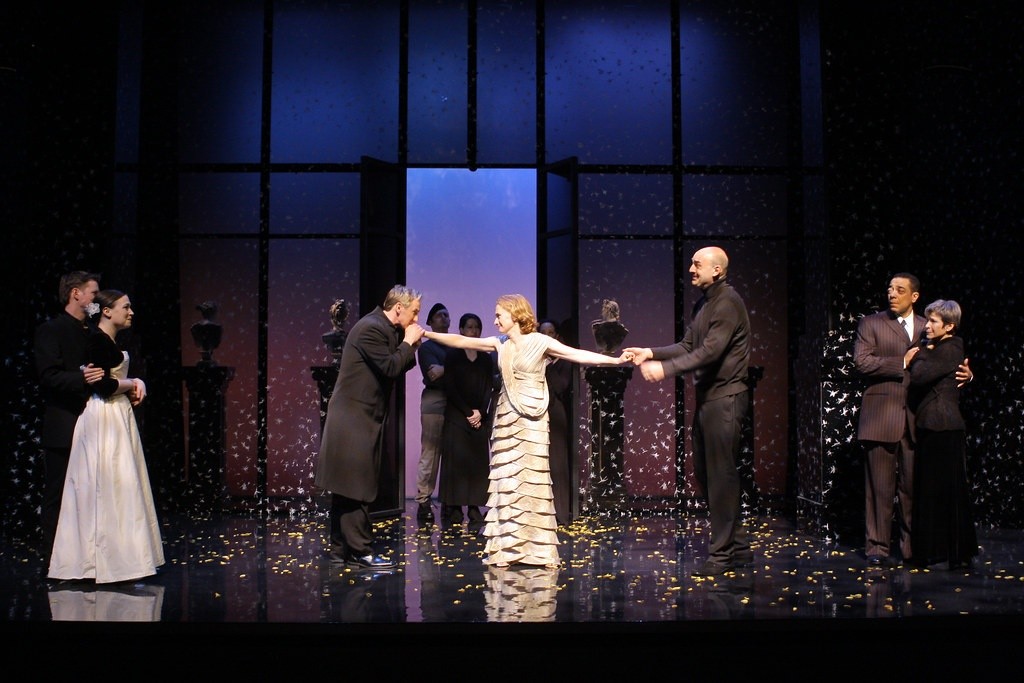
[426,303,446,325]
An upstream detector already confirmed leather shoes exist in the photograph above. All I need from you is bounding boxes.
[690,564,734,577]
[869,554,886,565]
[417,501,433,519]
[346,552,399,568]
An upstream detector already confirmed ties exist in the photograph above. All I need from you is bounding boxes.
[900,320,907,329]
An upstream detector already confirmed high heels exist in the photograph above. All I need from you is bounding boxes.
[467,505,486,523]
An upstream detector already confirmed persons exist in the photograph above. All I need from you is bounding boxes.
[417,299,628,530]
[34,272,168,586]
[853,274,980,568]
[624,245,755,577]
[48,530,995,623]
[315,284,425,567]
[423,294,635,566]
